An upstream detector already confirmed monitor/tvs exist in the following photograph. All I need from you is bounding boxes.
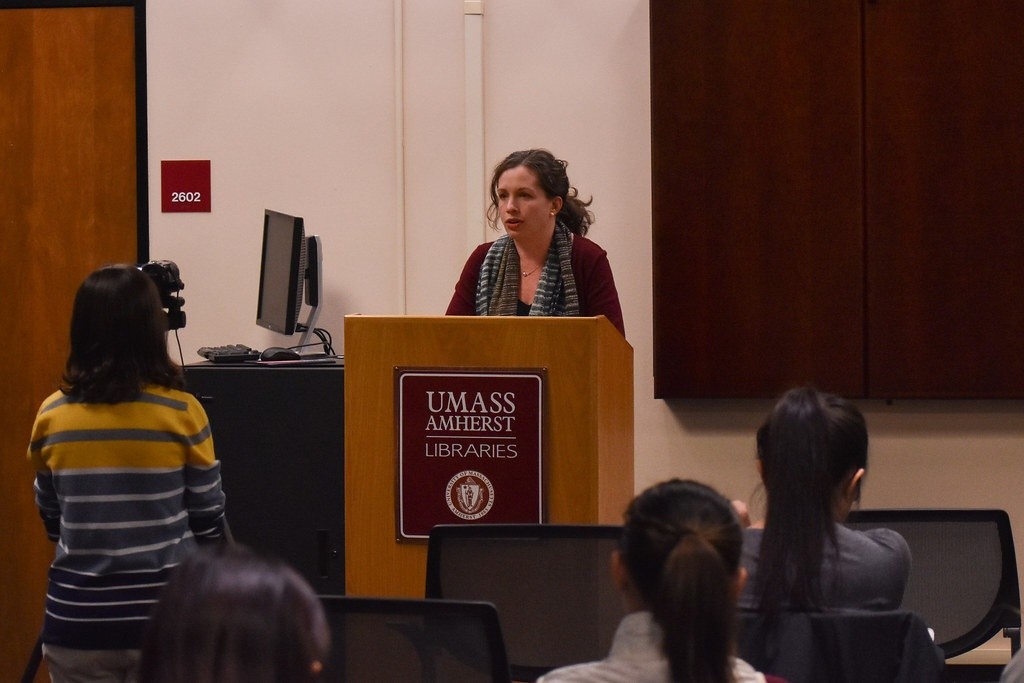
[255,209,328,358]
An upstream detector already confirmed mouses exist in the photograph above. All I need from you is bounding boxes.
[261,347,301,361]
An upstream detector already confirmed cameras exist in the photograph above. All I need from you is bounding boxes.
[137,259,184,298]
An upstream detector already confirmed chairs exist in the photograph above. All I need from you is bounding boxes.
[841,508,1022,683]
[734,608,949,683]
[314,595,511,683]
[425,525,635,683]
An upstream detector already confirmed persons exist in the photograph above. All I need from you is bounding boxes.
[537,479,792,683]
[731,389,911,623]
[135,553,328,683]
[445,148,626,339]
[27,268,229,683]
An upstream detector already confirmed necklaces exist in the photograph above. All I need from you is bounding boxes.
[520,260,546,277]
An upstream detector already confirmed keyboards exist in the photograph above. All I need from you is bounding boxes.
[197,344,260,363]
